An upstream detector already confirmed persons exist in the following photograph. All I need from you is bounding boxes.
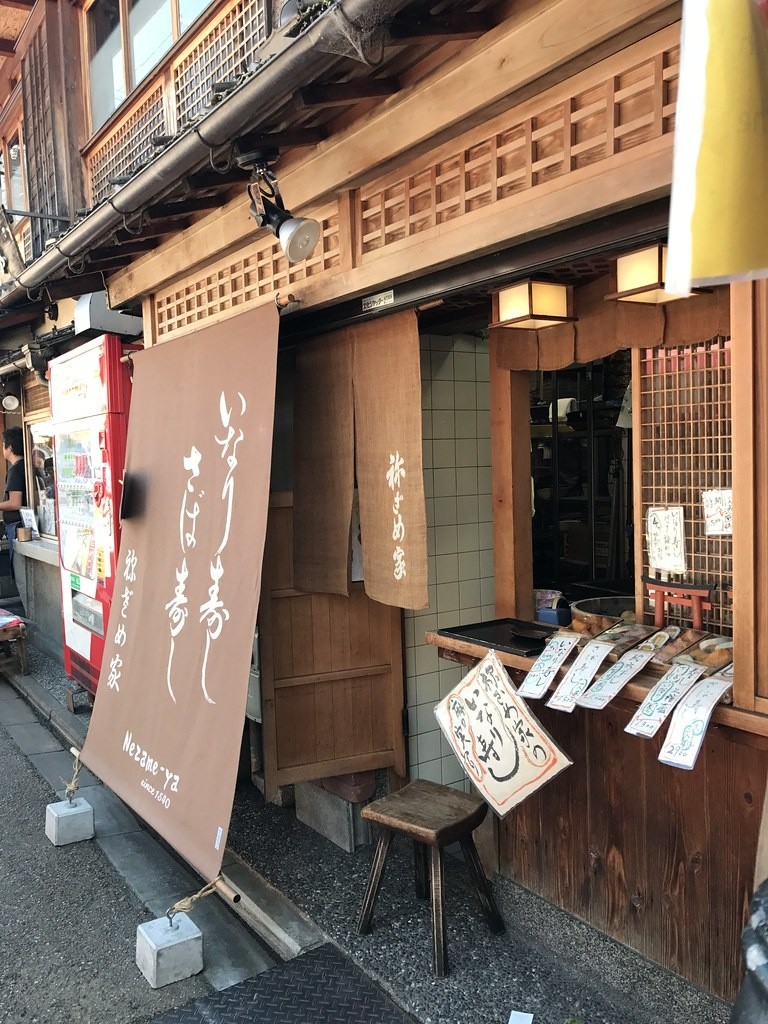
[31,449,55,499]
[0,426,47,584]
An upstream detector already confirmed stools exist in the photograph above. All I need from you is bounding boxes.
[357,778,505,980]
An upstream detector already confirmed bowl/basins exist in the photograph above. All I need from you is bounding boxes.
[508,626,555,643]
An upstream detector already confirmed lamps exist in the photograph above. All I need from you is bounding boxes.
[489,278,581,332]
[0,382,20,411]
[601,242,712,305]
[237,149,319,261]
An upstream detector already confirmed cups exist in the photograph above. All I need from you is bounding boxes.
[17,527,32,542]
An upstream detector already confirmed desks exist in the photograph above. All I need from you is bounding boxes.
[0,607,29,675]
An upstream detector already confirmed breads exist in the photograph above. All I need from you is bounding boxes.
[559,610,634,638]
[689,647,733,666]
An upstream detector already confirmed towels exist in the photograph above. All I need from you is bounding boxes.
[615,378,632,428]
[547,396,577,422]
